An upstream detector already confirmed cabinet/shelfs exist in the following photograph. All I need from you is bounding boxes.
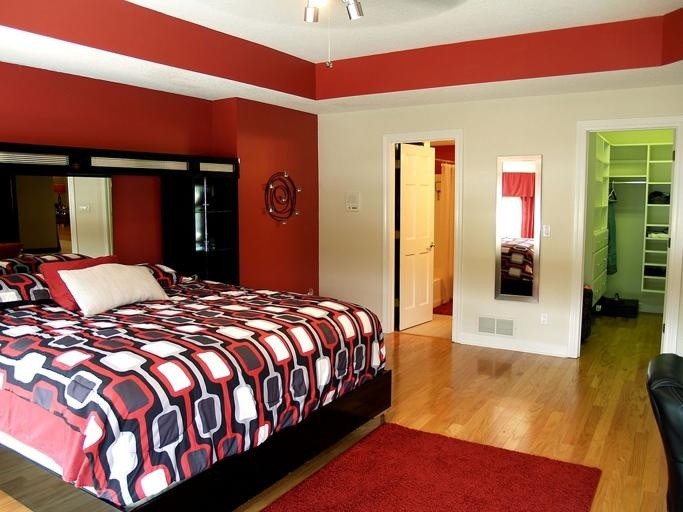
[583,128,675,307]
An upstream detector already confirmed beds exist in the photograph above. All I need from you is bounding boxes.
[500,236,535,296]
[0,273,394,512]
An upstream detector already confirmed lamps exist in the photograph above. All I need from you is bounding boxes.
[302,0,364,23]
[52,183,67,206]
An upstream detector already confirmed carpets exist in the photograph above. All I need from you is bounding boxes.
[259,422,603,512]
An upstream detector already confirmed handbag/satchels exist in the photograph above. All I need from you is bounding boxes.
[595,293,640,318]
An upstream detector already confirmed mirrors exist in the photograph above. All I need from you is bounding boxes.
[494,154,543,302]
[0,174,162,273]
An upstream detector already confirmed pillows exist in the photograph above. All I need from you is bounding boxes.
[0,254,177,320]
